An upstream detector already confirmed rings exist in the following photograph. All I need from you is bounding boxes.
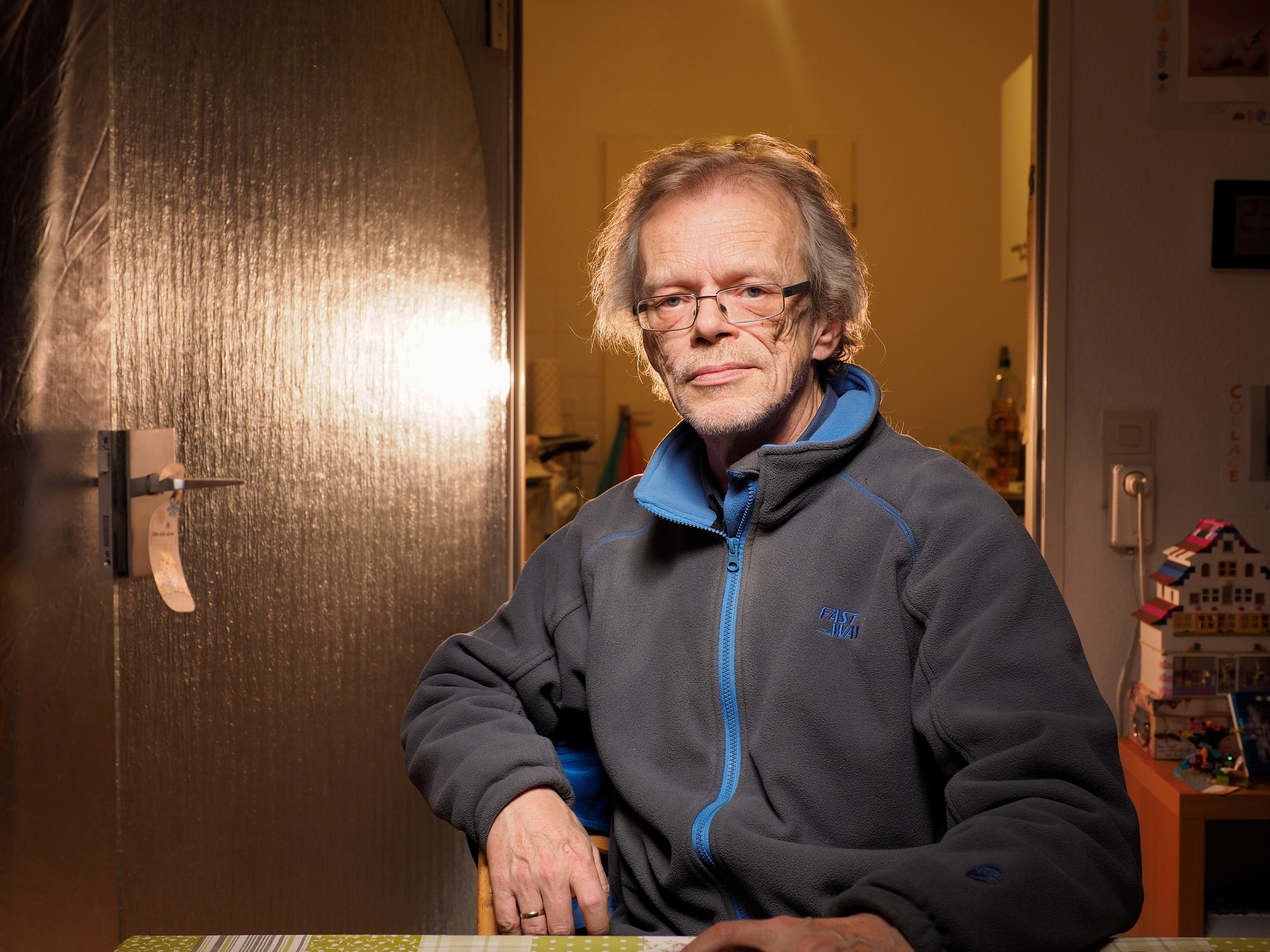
[518,909,544,920]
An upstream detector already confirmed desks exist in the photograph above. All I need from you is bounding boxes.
[1121,732,1270,938]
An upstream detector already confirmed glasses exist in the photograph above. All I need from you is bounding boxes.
[632,280,812,331]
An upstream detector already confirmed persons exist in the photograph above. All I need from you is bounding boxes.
[402,129,1143,951]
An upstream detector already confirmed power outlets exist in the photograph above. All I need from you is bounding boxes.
[1111,465,1154,552]
